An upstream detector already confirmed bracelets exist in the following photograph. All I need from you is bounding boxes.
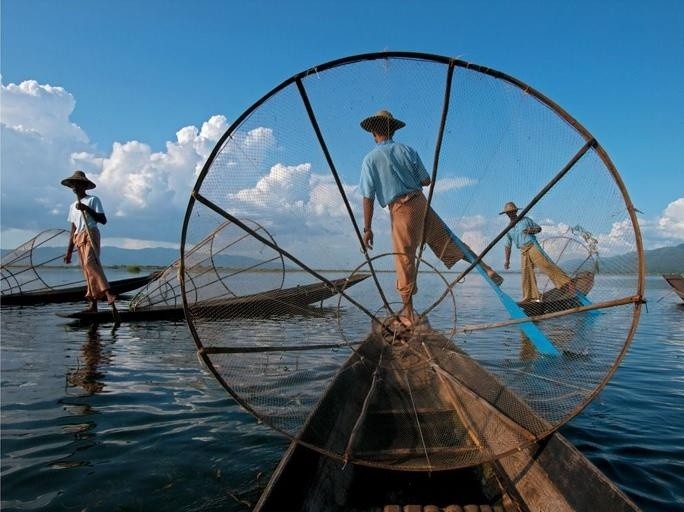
[363,228,371,232]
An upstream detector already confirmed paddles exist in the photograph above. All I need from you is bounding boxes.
[441,219,560,356]
[528,234,603,315]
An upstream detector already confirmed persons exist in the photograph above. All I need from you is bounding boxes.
[60,171,116,312]
[360,111,504,328]
[499,202,574,306]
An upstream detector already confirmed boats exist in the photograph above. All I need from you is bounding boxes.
[55,217,372,322]
[174,50,647,511]
[663,273,683,304]
[514,234,598,312]
[0,227,160,304]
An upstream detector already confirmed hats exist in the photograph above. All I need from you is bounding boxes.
[499,202,523,215]
[61,171,96,190]
[360,111,406,132]
